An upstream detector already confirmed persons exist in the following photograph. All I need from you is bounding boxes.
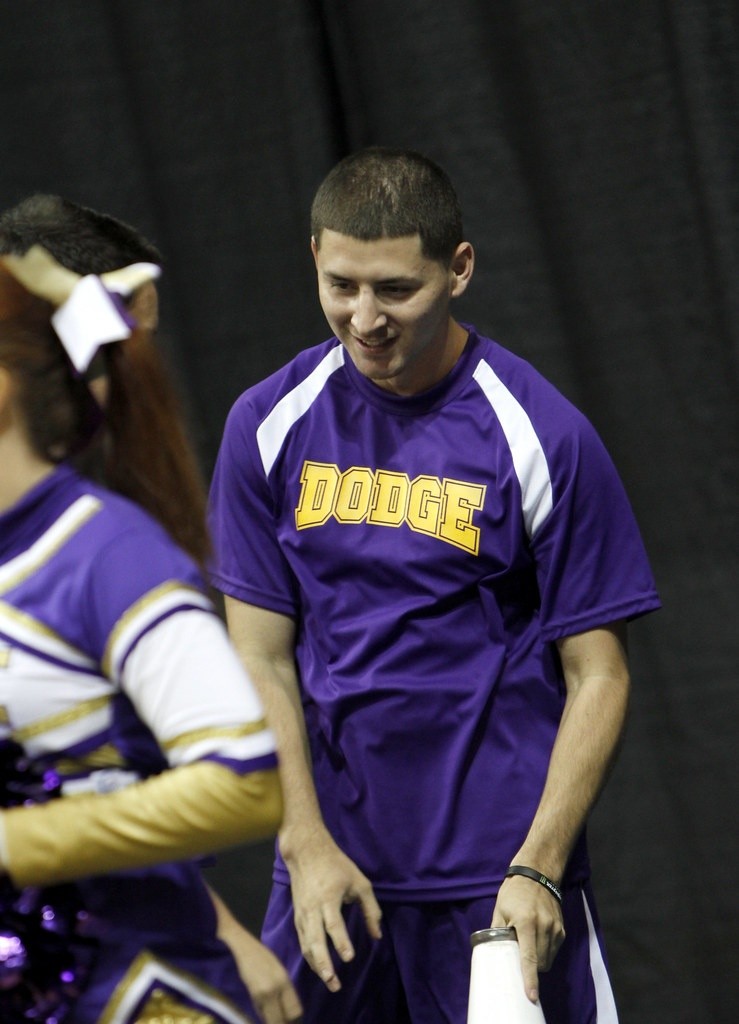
[204,147,661,1024]
[0,196,301,1024]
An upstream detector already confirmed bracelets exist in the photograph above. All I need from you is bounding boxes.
[505,866,562,901]
